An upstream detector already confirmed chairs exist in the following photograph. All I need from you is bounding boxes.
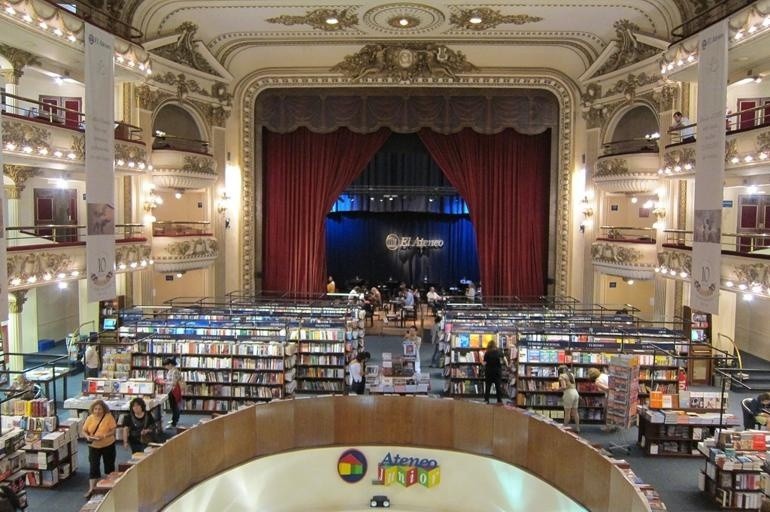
[363,283,447,330]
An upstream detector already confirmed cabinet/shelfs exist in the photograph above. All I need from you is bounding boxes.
[432,295,692,436]
[360,351,432,396]
[1,279,363,511]
[83,394,667,511]
[570,340,770,511]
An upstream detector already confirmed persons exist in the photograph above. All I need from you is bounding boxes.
[326,276,335,301]
[346,282,422,318]
[426,286,447,312]
[669,111,695,143]
[726,108,732,132]
[465,282,475,303]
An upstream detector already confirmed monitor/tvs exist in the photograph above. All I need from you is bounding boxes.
[103,319,117,331]
[691,329,704,343]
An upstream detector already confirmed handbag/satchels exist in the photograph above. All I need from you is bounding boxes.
[138,429,154,445]
[351,378,364,393]
[85,439,93,444]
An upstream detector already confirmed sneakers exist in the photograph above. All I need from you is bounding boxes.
[83,491,91,498]
[166,420,175,429]
